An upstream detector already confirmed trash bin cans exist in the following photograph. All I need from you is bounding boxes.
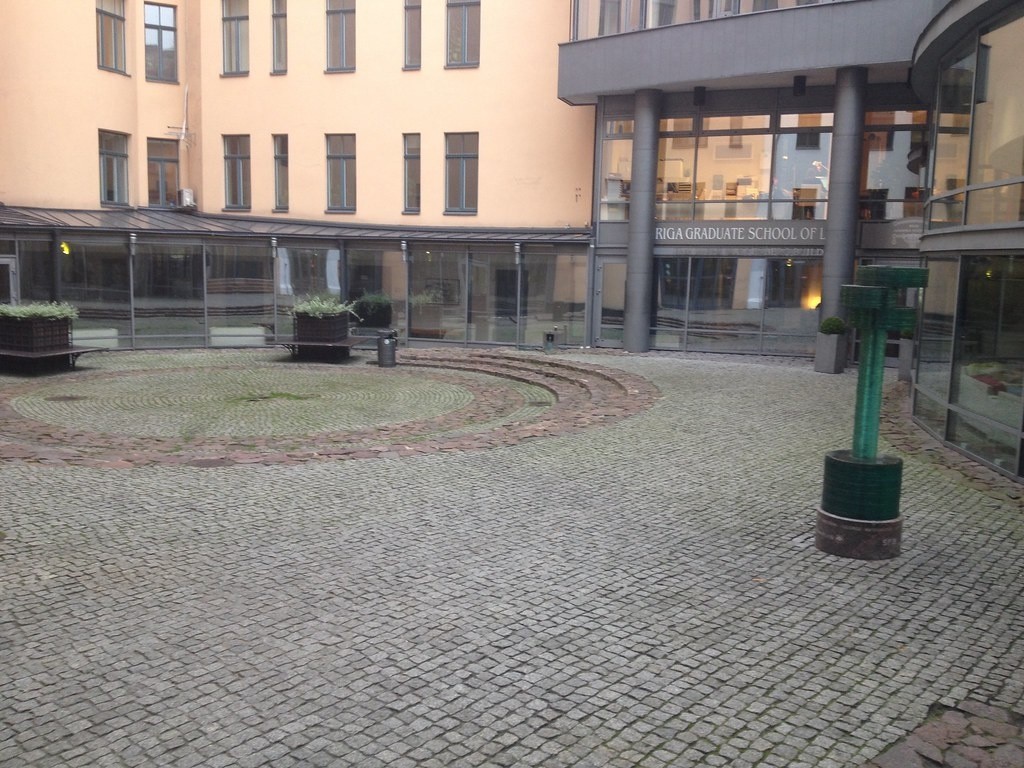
[543,330,559,356]
[376,329,397,368]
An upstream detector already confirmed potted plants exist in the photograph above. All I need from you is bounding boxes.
[353,290,393,329]
[812,316,849,374]
[0,298,79,374]
[409,290,447,340]
[288,293,356,360]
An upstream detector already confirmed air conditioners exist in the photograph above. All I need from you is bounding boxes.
[177,188,195,207]
